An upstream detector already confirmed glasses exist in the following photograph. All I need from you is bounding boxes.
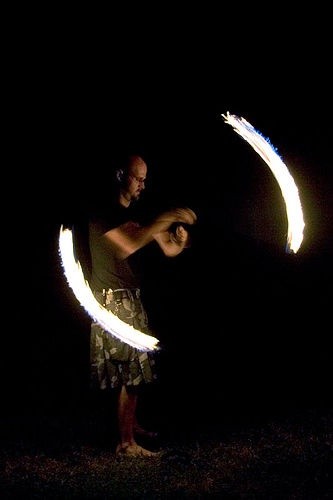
[129,174,147,182]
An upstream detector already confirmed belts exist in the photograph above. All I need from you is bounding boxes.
[114,288,142,300]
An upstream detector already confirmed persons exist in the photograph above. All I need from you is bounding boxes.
[86,153,196,460]
